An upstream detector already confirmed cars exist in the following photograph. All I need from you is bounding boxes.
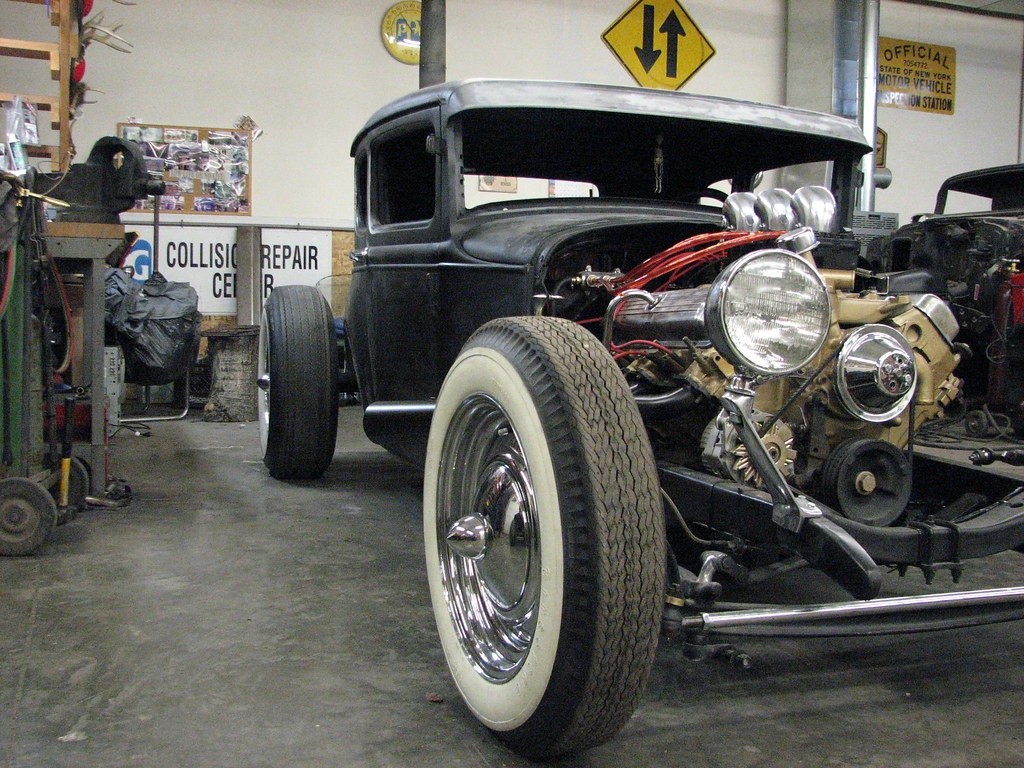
[255,79,1023,757]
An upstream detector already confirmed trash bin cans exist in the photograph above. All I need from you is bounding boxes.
[200,322,260,422]
